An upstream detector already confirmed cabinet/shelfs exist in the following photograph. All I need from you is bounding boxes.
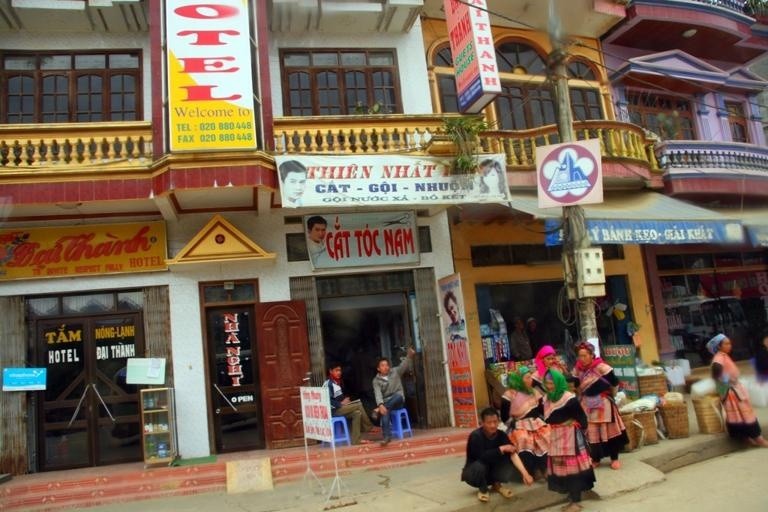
[140,388,175,470]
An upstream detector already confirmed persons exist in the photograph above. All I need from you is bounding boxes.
[306,216,329,267]
[499,364,550,483]
[477,160,507,201]
[322,362,373,445]
[459,407,534,504]
[441,291,465,333]
[280,160,308,207]
[565,342,630,469]
[755,334,768,374]
[368,343,417,447]
[541,367,597,511]
[509,315,533,360]
[706,333,768,448]
[525,317,543,357]
[530,344,566,396]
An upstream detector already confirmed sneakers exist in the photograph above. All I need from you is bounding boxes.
[592,458,621,470]
[560,503,585,512]
[738,434,767,448]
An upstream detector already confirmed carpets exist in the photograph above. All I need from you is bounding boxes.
[174,455,217,466]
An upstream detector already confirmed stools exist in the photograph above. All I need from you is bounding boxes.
[379,408,412,439]
[321,416,351,450]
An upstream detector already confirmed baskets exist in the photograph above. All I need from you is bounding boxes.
[618,394,724,453]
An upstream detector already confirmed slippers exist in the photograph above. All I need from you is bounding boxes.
[477,488,490,503]
[496,485,514,499]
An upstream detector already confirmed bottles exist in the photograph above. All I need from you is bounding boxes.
[144,422,169,433]
[149,440,168,459]
